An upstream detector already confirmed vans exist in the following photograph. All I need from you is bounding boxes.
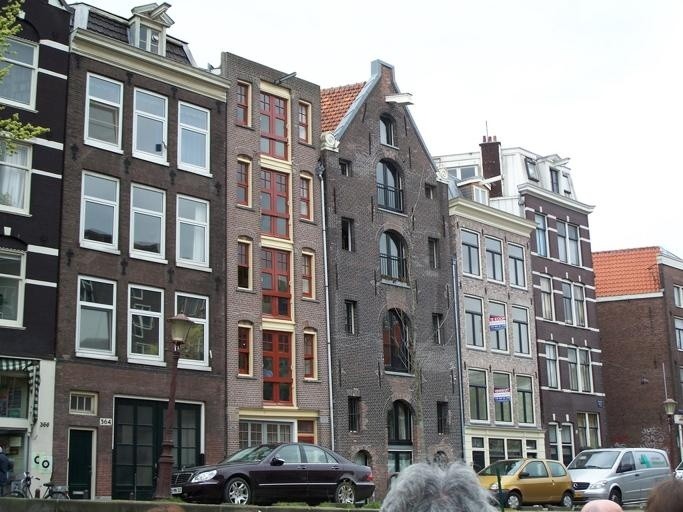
[566,447,672,508]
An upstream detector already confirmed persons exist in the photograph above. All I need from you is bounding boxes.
[643,478,682,511]
[377,460,500,512]
[0,446,14,497]
[582,499,623,511]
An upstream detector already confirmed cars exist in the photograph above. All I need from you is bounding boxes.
[476,458,574,509]
[171,442,375,505]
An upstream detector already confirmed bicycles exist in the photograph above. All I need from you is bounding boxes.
[3,472,71,499]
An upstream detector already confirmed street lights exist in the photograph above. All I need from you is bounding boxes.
[153,310,195,499]
[663,398,678,469]
